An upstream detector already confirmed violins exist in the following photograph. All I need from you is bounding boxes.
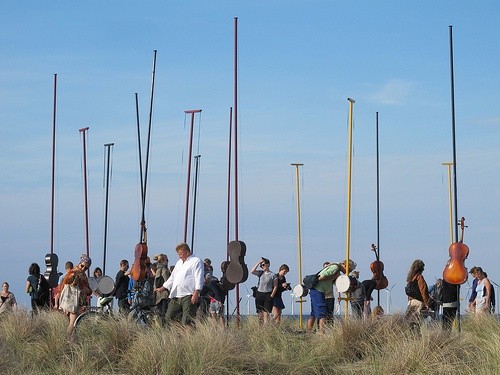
[370,244,388,290]
[443,217,470,284]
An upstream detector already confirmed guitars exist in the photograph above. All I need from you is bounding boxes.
[44,253,59,287]
[225,241,249,284]
[220,261,235,290]
[131,221,148,282]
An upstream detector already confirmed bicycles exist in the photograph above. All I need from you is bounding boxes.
[73,288,168,342]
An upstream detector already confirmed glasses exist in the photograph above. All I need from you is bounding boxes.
[260,263,265,267]
[80,263,88,270]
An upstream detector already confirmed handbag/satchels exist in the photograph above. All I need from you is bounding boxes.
[405,275,430,302]
[251,286,258,298]
[156,265,170,288]
[59,281,81,313]
[33,273,46,306]
[303,274,319,287]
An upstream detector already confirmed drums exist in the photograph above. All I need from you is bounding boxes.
[96,276,115,297]
[73,311,104,342]
[294,284,308,298]
[336,275,357,293]
[87,277,99,291]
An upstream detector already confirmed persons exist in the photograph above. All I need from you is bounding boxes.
[307,259,367,334]
[406,260,444,328]
[0,242,216,336]
[348,271,381,318]
[251,257,292,324]
[466,266,495,314]
